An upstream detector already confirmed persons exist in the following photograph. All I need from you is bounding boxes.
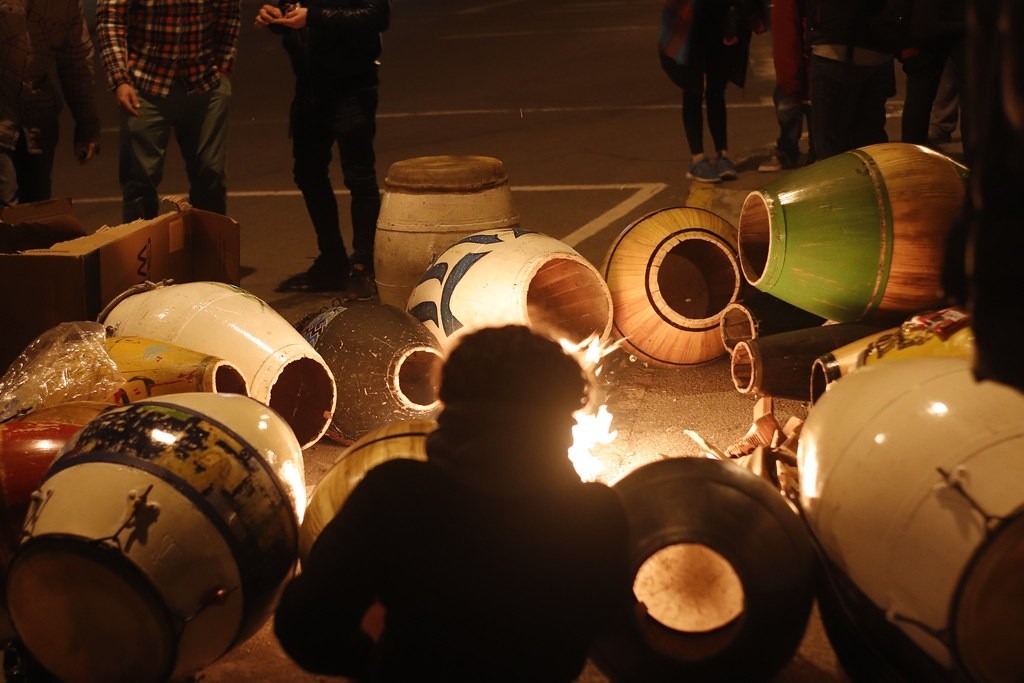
[255,0,390,300]
[95,0,241,224]
[273,324,633,683]
[658,0,1023,393]
[0,0,100,208]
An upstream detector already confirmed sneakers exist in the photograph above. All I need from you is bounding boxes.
[714,156,738,180]
[687,157,722,183]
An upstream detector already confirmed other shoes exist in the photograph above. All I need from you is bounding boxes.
[758,153,800,172]
[338,256,377,300]
[280,255,351,292]
[928,128,953,143]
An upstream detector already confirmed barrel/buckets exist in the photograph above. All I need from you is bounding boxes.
[3,156,1022,681]
